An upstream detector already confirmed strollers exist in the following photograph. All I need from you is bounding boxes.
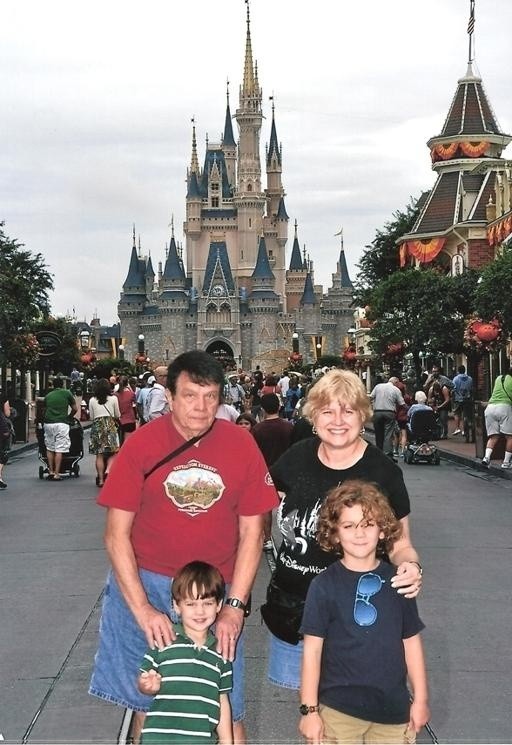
[34,416,84,479]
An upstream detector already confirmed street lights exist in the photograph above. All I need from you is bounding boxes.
[119,343,124,361]
[138,333,145,355]
[316,343,322,360]
[79,329,93,394]
[292,331,300,354]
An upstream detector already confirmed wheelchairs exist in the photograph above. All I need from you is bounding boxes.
[400,409,443,465]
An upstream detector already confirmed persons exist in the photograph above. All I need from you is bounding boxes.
[137,561,232,745]
[296,479,431,744]
[261,369,423,691]
[87,350,280,745]
[0,360,512,487]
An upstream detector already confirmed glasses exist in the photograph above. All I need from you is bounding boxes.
[354,573,386,627]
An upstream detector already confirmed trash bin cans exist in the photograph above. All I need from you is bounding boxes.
[474,400,512,460]
[36,397,45,418]
[2,398,29,444]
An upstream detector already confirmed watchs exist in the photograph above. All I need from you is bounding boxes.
[300,704,318,716]
[225,598,250,618]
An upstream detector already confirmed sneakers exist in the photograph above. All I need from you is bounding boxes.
[452,428,464,436]
[481,457,512,469]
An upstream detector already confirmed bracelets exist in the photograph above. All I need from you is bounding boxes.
[409,561,422,576]
